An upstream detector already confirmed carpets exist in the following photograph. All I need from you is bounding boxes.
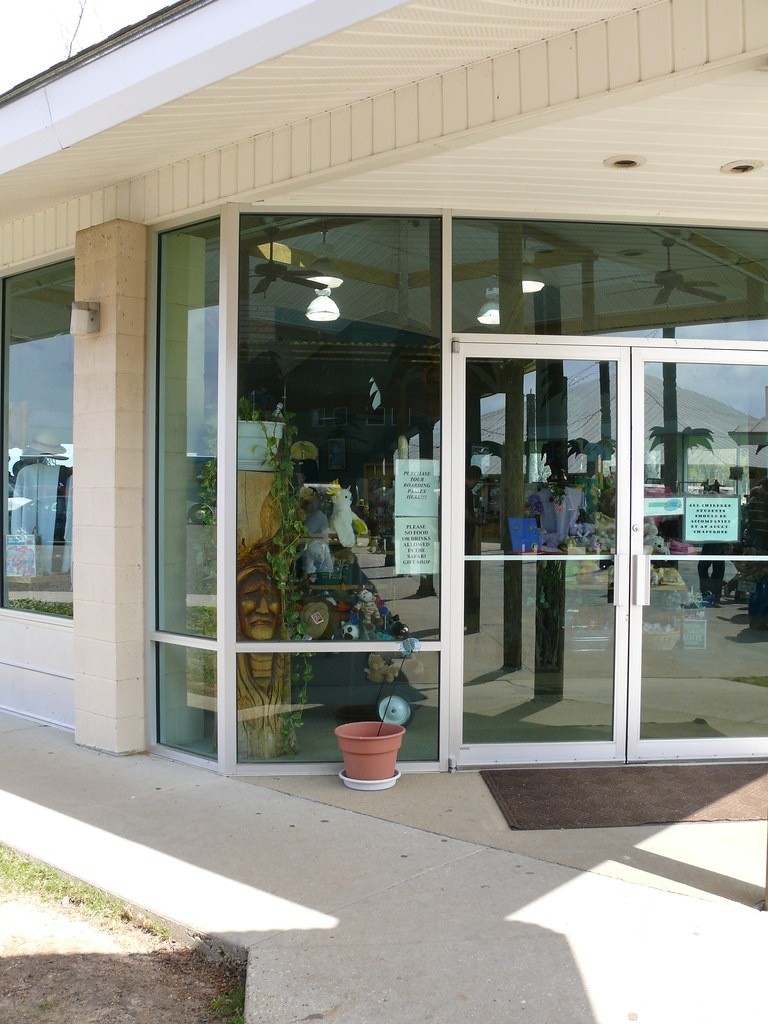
[478,762,768,832]
[461,717,731,744]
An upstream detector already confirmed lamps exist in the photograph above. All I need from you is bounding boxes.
[476,275,500,324]
[305,230,344,288]
[305,289,341,322]
[521,236,547,293]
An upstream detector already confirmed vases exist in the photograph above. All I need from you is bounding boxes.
[336,722,407,780]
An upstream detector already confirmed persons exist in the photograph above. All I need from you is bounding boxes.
[697,478,728,607]
[465,465,483,606]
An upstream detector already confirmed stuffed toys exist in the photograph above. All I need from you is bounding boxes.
[569,512,616,551]
[298,478,368,572]
[645,524,682,582]
[365,652,399,683]
[299,588,414,642]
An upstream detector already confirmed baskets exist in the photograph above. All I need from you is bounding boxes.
[642,630,678,651]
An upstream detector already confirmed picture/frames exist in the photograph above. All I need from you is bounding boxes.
[327,438,346,470]
[311,407,326,428]
[366,406,386,427]
[392,407,411,427]
[332,406,348,428]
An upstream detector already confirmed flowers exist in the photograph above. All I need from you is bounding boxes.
[535,467,566,511]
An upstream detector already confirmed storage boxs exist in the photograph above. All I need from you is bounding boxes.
[238,417,287,472]
[674,619,707,651]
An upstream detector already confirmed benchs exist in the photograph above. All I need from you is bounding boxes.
[607,565,689,629]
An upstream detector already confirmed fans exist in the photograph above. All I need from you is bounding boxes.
[203,227,329,297]
[606,239,728,305]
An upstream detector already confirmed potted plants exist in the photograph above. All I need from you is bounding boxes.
[565,536,586,576]
[185,383,313,756]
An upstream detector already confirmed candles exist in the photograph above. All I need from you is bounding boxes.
[284,377,287,397]
[250,390,255,412]
